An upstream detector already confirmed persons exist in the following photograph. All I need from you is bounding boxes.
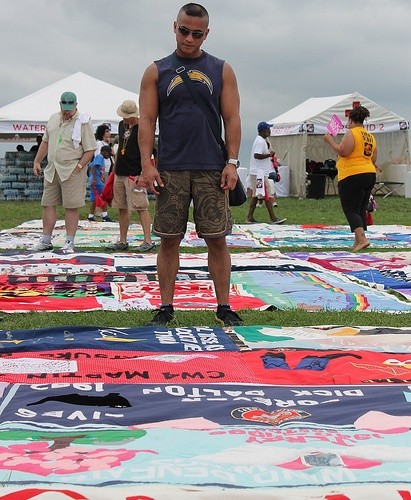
[105,100,157,253]
[134,3,246,326]
[16,145,26,151]
[323,105,378,254]
[26,91,99,254]
[87,121,122,222]
[243,122,287,225]
[29,135,42,152]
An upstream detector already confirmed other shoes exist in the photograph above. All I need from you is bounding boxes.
[148,195,155,200]
[274,218,287,224]
[130,242,156,253]
[87,217,95,221]
[272,202,277,207]
[102,215,115,222]
[247,219,257,223]
[59,241,74,253]
[256,204,262,207]
[35,240,54,253]
[106,242,129,252]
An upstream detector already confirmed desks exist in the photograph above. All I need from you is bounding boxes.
[236,168,249,193]
[275,164,290,198]
[372,181,405,199]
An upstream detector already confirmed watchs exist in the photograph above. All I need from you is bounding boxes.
[227,159,240,168]
[77,164,84,168]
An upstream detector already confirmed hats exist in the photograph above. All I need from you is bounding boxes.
[101,146,115,156]
[258,122,273,132]
[60,92,77,110]
[117,100,139,118]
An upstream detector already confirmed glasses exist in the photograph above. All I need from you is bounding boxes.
[105,131,109,132]
[178,26,204,38]
[61,101,74,104]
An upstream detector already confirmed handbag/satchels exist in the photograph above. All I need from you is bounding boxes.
[102,172,114,203]
[94,190,105,208]
[366,211,373,226]
[230,176,247,207]
[108,163,113,174]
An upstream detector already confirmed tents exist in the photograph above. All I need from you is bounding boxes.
[260,92,411,199]
[0,73,160,151]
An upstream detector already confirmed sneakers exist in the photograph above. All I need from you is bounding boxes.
[215,305,244,326]
[151,304,175,325]
